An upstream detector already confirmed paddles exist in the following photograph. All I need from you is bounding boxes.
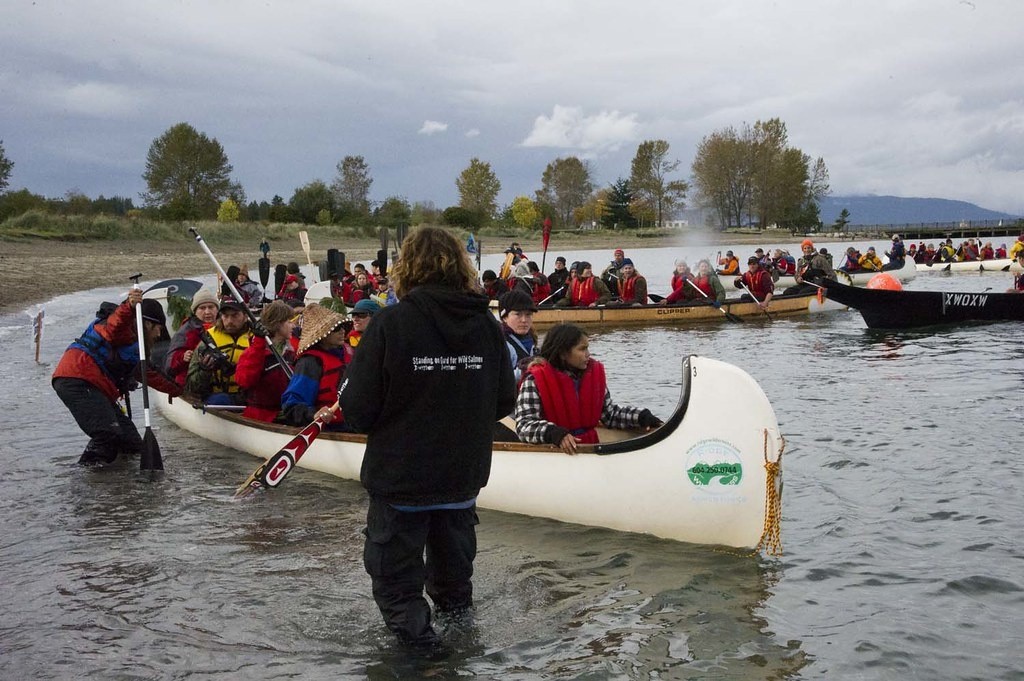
[606,271,677,304]
[128,273,164,471]
[800,237,1021,293]
[737,279,773,320]
[477,240,481,285]
[538,286,565,306]
[298,230,317,284]
[379,228,389,250]
[716,251,721,274]
[259,258,271,297]
[326,248,389,306]
[391,251,399,264]
[502,252,515,281]
[397,223,408,248]
[239,401,340,490]
[193,402,247,409]
[188,226,294,379]
[542,217,552,275]
[394,240,398,252]
[686,278,744,323]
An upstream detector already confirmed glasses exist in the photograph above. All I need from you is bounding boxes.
[351,313,371,319]
[331,322,347,332]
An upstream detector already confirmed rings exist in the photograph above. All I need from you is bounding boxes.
[318,413,327,418]
[328,408,331,411]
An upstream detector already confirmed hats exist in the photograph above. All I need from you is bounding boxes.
[755,248,763,252]
[623,258,633,266]
[296,303,352,355]
[869,246,875,251]
[675,257,686,266]
[189,285,220,315]
[348,298,381,315]
[919,234,1024,250]
[215,296,243,320]
[614,248,624,257]
[801,239,813,252]
[482,270,495,281]
[893,234,899,240]
[504,247,515,254]
[910,244,915,248]
[141,298,166,327]
[556,257,566,266]
[570,261,579,270]
[748,256,758,264]
[238,264,249,277]
[498,290,538,319]
[726,251,733,256]
[284,276,298,284]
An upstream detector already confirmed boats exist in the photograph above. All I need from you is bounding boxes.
[913,254,1020,272]
[796,266,1024,331]
[123,278,789,563]
[488,268,856,324]
[713,252,919,292]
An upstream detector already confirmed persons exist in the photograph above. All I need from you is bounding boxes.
[516,324,665,456]
[162,288,383,433]
[906,232,1024,295]
[660,234,907,310]
[221,241,648,308]
[337,225,517,645]
[53,289,183,466]
[498,291,542,410]
